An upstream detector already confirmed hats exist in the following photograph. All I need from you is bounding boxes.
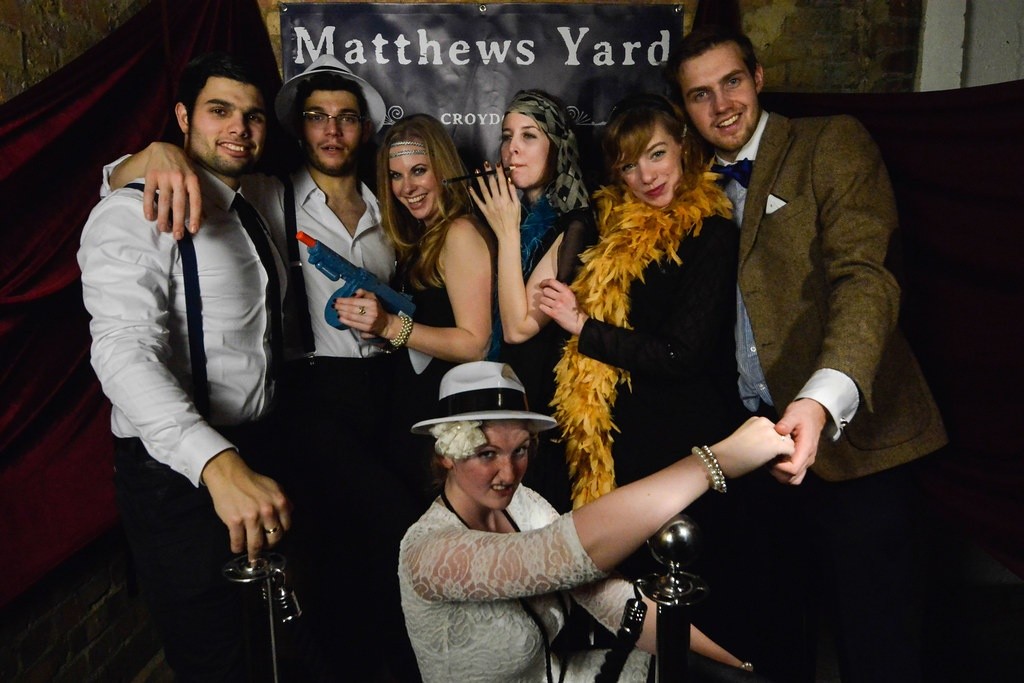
[274,55,386,136]
[409,359,556,433]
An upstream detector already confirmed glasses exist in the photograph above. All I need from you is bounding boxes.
[300,110,365,131]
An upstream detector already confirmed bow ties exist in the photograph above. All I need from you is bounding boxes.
[711,158,753,190]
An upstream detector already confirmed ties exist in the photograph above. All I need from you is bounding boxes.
[232,192,283,380]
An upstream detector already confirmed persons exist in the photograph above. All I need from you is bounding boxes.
[98,56,425,683]
[672,24,950,683]
[398,361,794,683]
[467,87,599,520]
[333,111,501,514]
[76,53,289,683]
[537,93,733,516]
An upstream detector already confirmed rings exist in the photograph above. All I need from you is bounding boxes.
[263,525,282,534]
[358,308,365,316]
[781,436,785,442]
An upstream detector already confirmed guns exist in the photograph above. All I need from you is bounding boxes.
[294,230,417,344]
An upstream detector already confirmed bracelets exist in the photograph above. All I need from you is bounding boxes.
[390,315,414,348]
[692,443,729,497]
[733,661,755,673]
